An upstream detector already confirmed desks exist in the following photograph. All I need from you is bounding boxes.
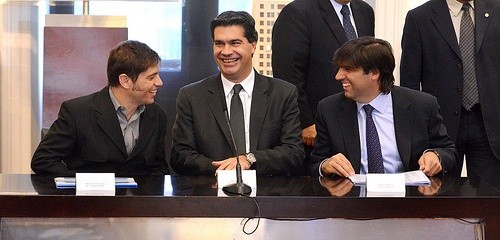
[1,170,500,240]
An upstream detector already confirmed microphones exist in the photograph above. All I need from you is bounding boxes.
[221,96,250,194]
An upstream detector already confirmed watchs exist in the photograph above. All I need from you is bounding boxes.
[425,150,440,162]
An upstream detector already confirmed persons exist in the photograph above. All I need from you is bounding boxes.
[308,36,459,177]
[272,0,374,162]
[170,10,306,177]
[31,41,169,175]
[400,0,500,192]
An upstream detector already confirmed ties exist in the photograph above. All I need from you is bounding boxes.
[229,84,247,156]
[339,4,357,42]
[459,3,478,109]
[362,104,384,174]
